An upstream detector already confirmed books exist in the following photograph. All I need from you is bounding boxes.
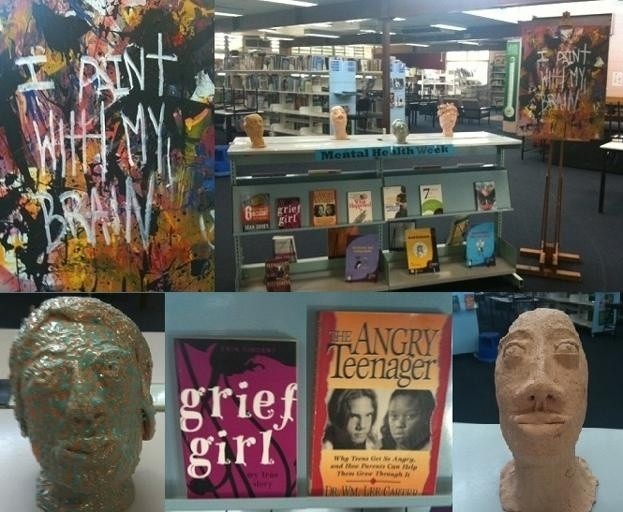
[217,50,382,135]
[307,304,452,499]
[488,51,506,109]
[165,330,300,499]
[243,177,499,292]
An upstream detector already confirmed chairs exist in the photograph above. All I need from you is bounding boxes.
[443,98,491,127]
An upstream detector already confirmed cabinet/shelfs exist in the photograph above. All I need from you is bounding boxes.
[215,67,384,136]
[230,133,522,293]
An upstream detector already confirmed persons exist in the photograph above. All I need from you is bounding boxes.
[493,307,599,512]
[322,389,377,450]
[7,295,158,512]
[389,118,410,145]
[381,386,434,454]
[329,104,352,142]
[435,102,460,137]
[242,112,266,149]
